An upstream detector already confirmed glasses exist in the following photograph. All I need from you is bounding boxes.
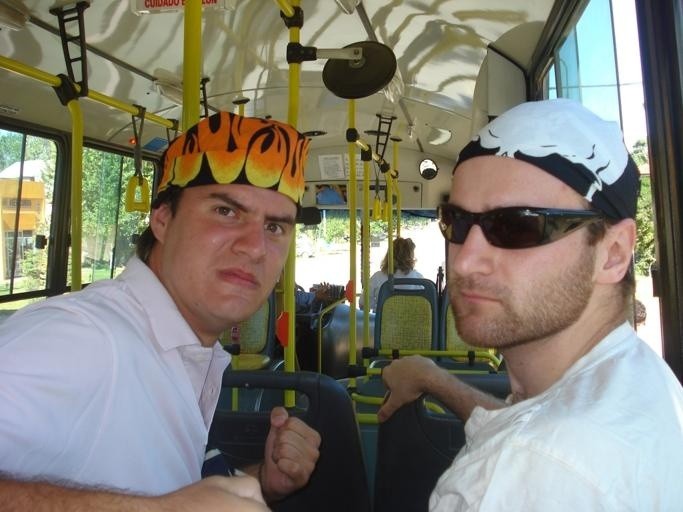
[435,201,601,251]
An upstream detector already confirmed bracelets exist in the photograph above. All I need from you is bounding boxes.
[256,459,267,487]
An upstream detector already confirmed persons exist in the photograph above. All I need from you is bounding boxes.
[378,98,683,512]
[293,281,331,329]
[358,237,427,314]
[0,109,323,512]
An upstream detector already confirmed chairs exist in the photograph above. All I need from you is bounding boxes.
[207,368,377,509]
[441,285,506,372]
[215,284,277,410]
[377,373,510,511]
[369,278,440,366]
